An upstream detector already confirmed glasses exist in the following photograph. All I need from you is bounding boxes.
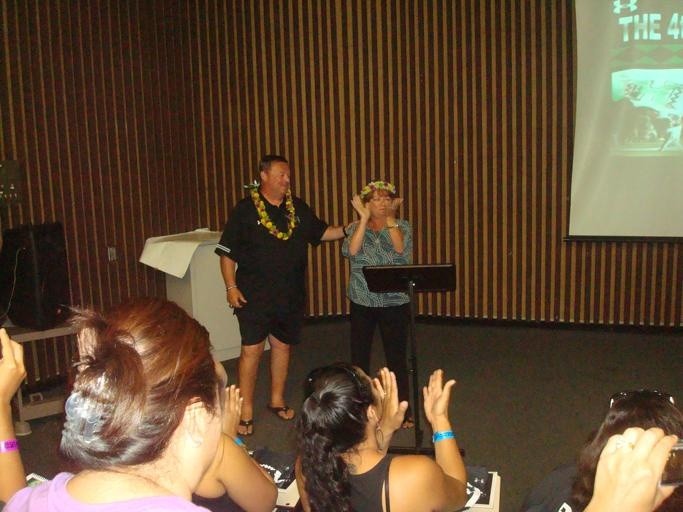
[609,391,674,409]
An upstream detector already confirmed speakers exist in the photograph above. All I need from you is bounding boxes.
[1,221,72,331]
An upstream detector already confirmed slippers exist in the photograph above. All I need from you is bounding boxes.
[267,403,295,420]
[237,420,254,436]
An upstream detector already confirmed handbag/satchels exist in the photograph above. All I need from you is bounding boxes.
[254,451,297,489]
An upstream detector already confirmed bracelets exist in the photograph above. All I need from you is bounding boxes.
[431,431,456,444]
[343,225,348,237]
[385,224,399,229]
[0,438,20,454]
[234,436,244,446]
[225,285,237,292]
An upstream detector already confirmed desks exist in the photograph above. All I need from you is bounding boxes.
[0,306,82,436]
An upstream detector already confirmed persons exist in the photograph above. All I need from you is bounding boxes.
[0,326,27,511]
[340,181,416,430]
[2,295,223,512]
[294,360,467,511]
[212,154,348,437]
[518,389,683,512]
[582,426,680,511]
[192,358,278,512]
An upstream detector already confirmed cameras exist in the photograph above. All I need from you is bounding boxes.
[658,439,683,487]
